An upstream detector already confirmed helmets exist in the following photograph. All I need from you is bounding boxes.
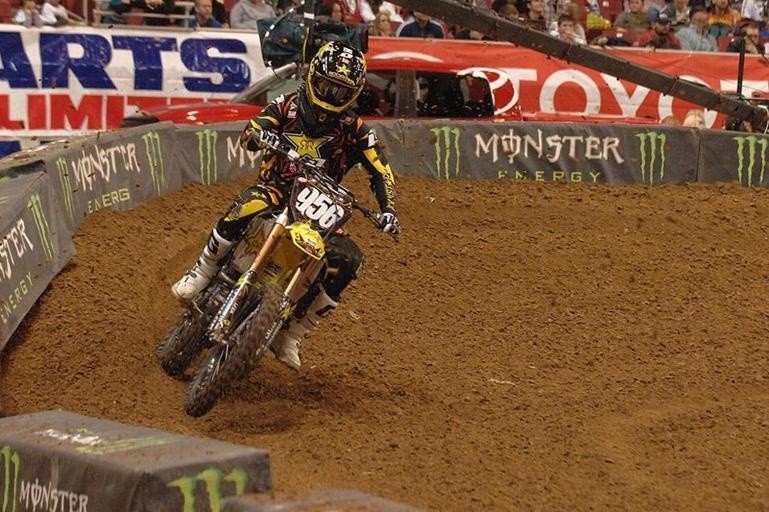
[306,40,366,124]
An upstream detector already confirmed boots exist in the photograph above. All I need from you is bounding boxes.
[271,283,341,371]
[171,228,239,300]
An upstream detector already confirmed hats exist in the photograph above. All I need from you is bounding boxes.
[657,14,672,25]
[689,6,712,17]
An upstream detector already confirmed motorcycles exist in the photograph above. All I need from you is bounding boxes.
[161,130,400,418]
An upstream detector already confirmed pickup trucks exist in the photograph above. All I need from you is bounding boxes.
[119,57,661,128]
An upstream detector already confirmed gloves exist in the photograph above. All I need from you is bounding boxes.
[378,213,402,237]
[255,131,280,152]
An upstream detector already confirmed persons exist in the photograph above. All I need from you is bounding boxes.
[1,0,767,56]
[172,41,402,373]
